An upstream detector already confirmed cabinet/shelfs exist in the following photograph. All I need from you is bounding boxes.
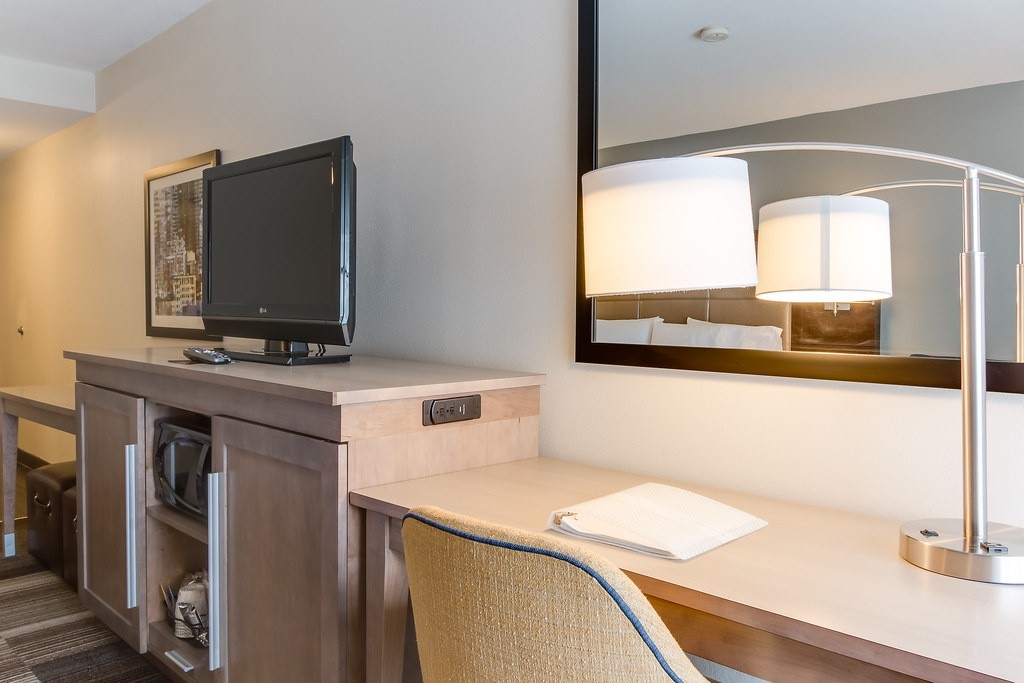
[63,343,547,683]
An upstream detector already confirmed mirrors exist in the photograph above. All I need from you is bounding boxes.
[574,0,1024,394]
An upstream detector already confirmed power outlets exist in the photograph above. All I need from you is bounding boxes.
[422,394,482,426]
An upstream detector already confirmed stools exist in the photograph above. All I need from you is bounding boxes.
[25,461,76,570]
[62,486,78,592]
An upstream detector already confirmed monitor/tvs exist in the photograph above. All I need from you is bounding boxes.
[200,134,356,366]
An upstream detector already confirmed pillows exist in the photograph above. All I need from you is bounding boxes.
[686,316,784,351]
[596,317,664,344]
[651,317,687,346]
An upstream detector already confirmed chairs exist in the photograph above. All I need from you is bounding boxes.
[400,503,711,683]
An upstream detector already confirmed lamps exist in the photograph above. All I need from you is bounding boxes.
[754,179,1024,363]
[582,140,1024,585]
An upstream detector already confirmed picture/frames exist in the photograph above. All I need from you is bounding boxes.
[144,148,224,342]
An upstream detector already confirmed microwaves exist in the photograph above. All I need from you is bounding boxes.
[154,419,212,526]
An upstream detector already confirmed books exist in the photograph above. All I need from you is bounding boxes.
[550,482,770,561]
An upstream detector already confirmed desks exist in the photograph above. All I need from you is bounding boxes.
[349,455,1024,683]
[0,383,75,558]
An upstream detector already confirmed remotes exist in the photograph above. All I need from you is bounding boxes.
[183,347,232,365]
